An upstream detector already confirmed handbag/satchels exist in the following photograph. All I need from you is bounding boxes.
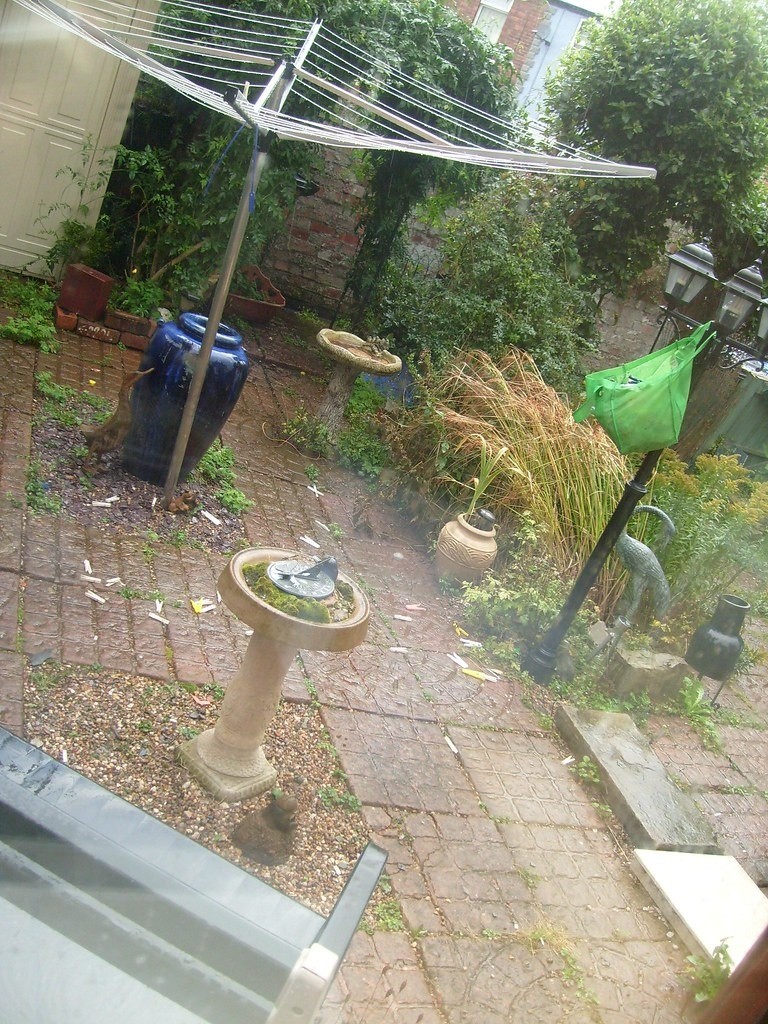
[570,320,718,454]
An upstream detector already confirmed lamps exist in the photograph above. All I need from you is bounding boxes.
[656,236,768,374]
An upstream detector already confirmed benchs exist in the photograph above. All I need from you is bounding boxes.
[0,724,389,1024]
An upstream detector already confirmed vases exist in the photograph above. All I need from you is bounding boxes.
[435,513,498,589]
[117,311,250,488]
[205,260,284,324]
